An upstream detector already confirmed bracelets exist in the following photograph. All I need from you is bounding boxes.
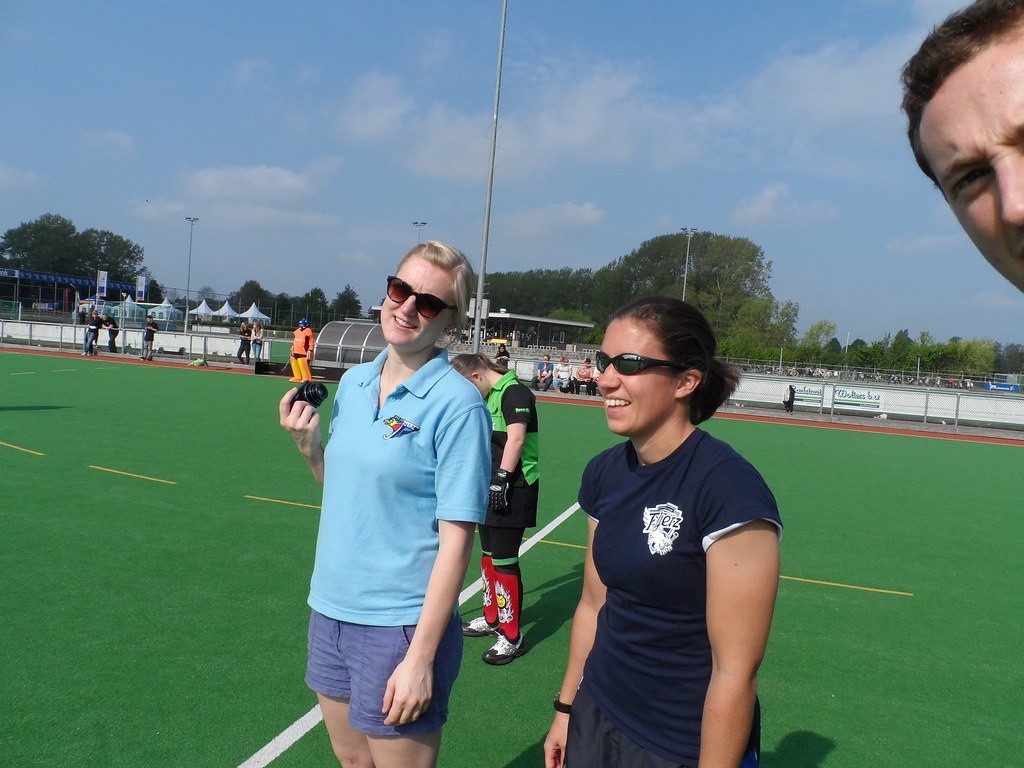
[554,692,571,714]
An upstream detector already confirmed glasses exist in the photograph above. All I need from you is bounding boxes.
[595,350,689,375]
[387,276,458,319]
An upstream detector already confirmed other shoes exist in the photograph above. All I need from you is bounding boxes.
[140,357,152,361]
[81,351,92,356]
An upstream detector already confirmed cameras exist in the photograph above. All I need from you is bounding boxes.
[290,380,328,408]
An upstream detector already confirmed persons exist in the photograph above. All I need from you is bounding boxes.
[494,344,509,368]
[553,357,572,393]
[78,309,119,357]
[279,240,492,768]
[592,366,601,396]
[781,385,794,414]
[140,316,159,361]
[532,354,553,390]
[449,352,540,665]
[732,363,973,389]
[572,358,592,395]
[540,294,786,768]
[288,318,315,382]
[236,321,261,364]
[898,0,1024,291]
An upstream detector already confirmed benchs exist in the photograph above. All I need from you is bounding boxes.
[520,379,600,395]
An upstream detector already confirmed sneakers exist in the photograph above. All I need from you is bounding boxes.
[482,631,526,665]
[461,617,499,636]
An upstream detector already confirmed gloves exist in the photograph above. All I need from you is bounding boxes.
[489,468,511,510]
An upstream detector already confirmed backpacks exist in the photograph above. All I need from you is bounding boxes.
[188,359,208,366]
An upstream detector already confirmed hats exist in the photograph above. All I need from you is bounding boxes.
[584,358,591,363]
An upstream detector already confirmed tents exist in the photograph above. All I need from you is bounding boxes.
[188,298,272,325]
[113,294,146,319]
[148,297,183,321]
[78,294,105,314]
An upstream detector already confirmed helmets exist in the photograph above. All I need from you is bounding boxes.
[299,318,308,328]
